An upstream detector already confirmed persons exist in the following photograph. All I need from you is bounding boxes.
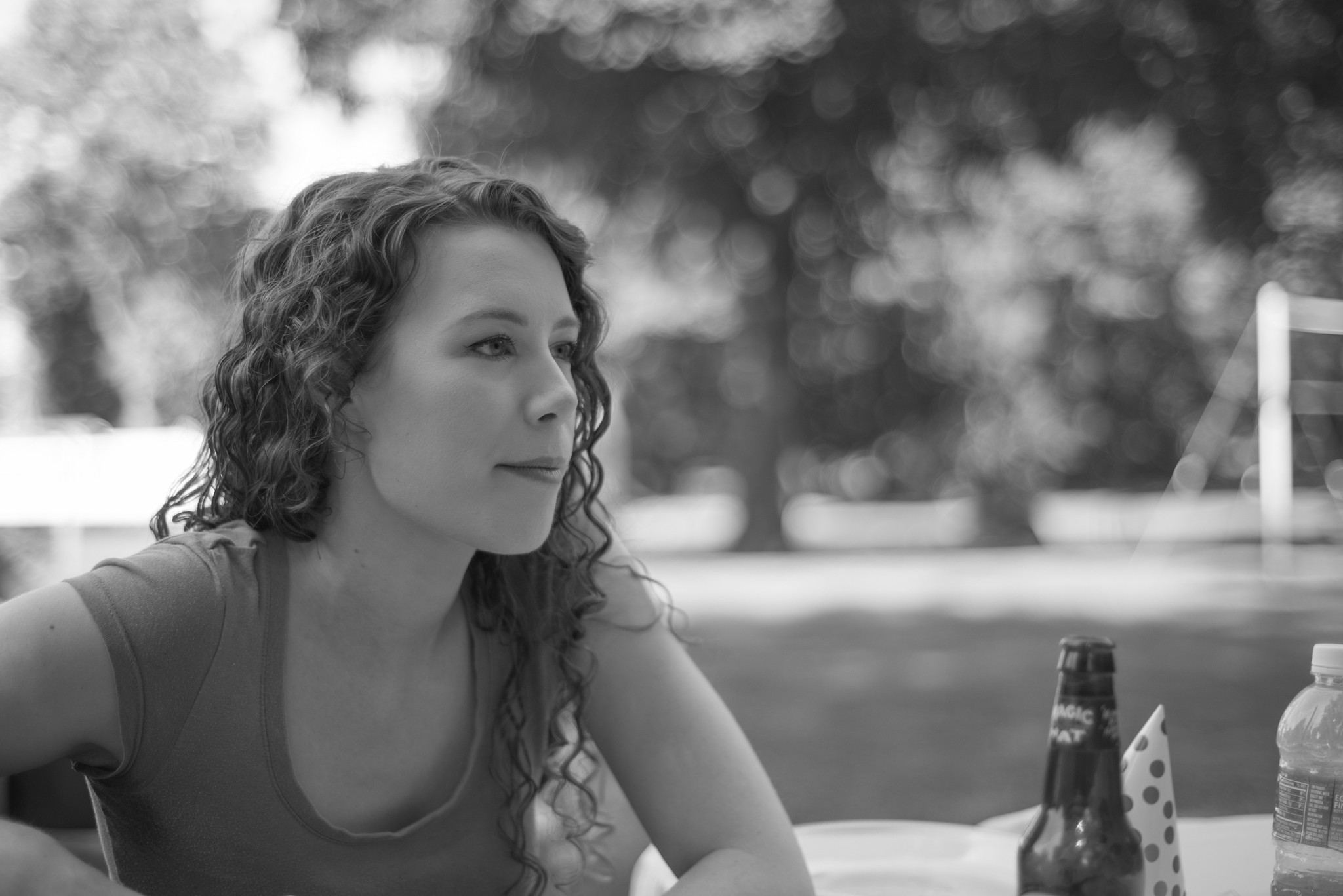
[0,156,814,896]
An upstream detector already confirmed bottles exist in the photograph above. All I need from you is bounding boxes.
[1270,643,1343,896]
[1018,636,1144,896]
